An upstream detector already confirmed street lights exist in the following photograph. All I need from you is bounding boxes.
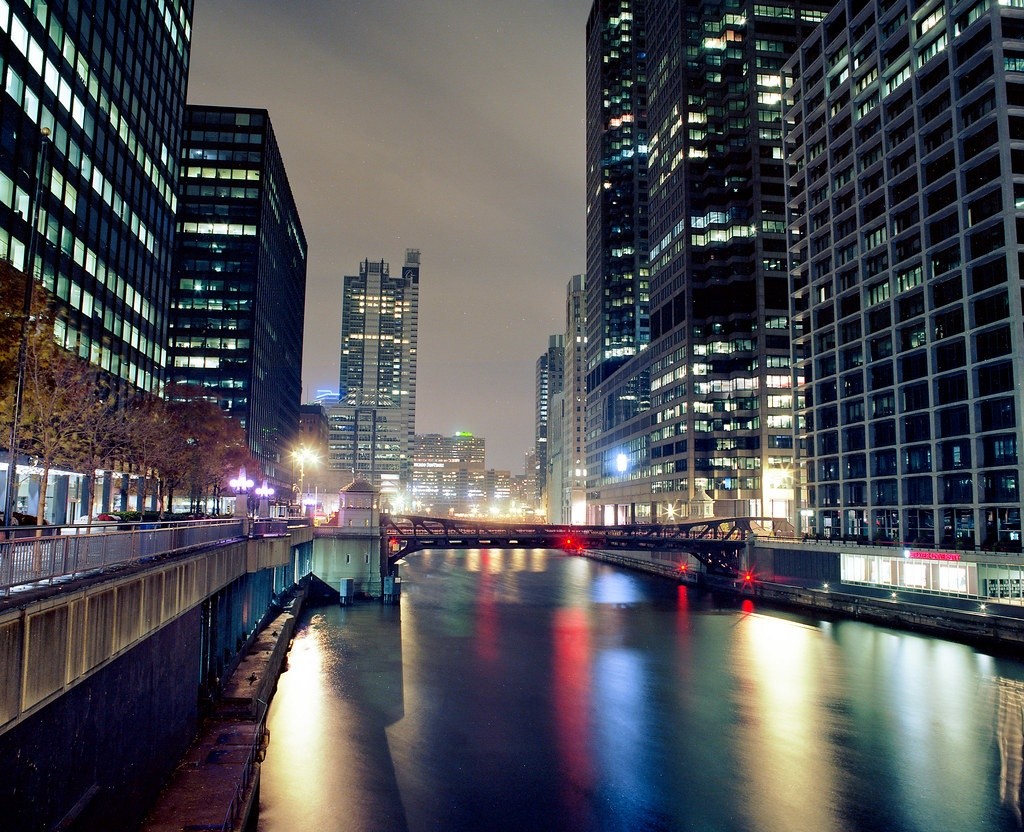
[296,447,318,517]
[229,475,254,494]
[256,486,274,498]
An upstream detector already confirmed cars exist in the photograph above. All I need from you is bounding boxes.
[0,511,61,553]
[59,514,123,536]
